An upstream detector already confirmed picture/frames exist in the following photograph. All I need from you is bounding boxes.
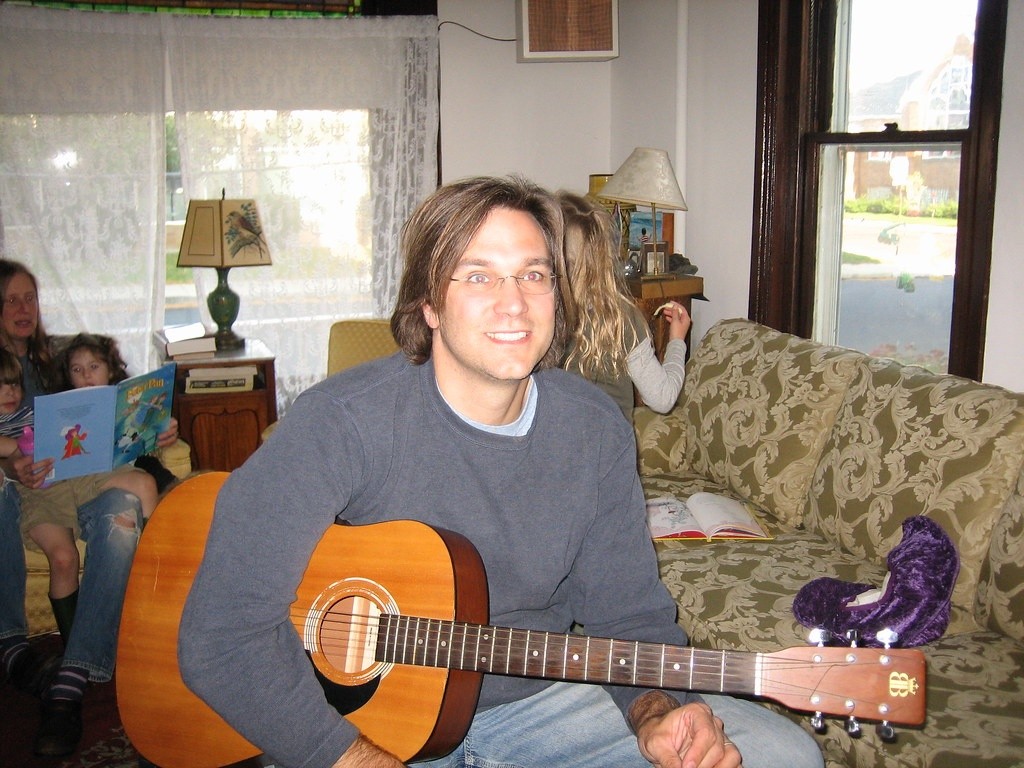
[641,242,669,275]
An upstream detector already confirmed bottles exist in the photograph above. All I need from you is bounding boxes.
[18,426,52,489]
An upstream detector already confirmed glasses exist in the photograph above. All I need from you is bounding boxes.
[450,265,562,297]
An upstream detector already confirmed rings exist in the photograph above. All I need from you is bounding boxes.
[724,742,733,746]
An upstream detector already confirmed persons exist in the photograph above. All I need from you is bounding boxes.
[555,190,690,428]
[176,178,823,768]
[0,257,179,757]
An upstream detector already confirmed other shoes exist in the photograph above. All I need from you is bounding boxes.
[10,646,58,695]
[48,588,78,647]
[37,696,83,756]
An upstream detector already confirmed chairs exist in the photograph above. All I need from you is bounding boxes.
[261,316,409,444]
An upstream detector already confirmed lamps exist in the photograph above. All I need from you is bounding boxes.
[179,190,270,351]
[597,147,689,278]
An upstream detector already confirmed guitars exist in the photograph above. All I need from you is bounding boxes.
[113,470,926,768]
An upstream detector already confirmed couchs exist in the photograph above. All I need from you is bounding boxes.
[634,321,1024,768]
[23,337,194,639]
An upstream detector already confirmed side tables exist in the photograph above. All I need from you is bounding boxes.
[161,339,276,475]
[623,275,708,405]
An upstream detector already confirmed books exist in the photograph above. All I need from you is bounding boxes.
[646,492,774,543]
[34,363,176,485]
[184,364,258,394]
[153,326,217,361]
